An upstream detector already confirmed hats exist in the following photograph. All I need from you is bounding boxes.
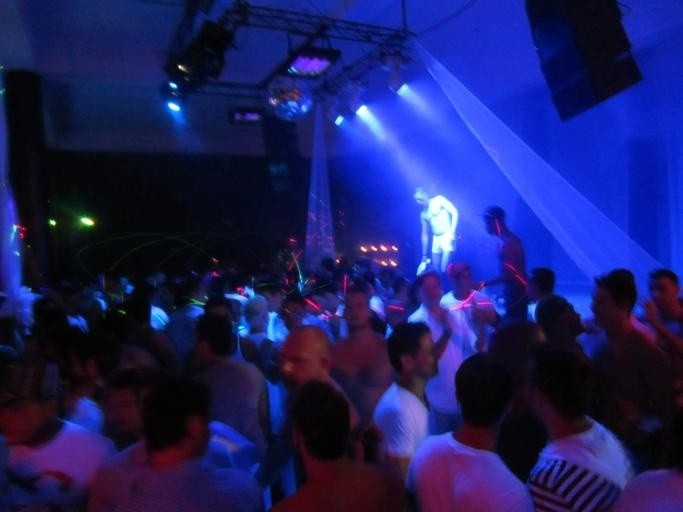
[477,203,505,220]
[0,358,61,405]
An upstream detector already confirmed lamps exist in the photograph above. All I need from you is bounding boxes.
[317,61,406,128]
[281,30,344,80]
[172,18,235,78]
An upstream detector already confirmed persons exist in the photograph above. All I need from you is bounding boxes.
[409,185,460,272]
[477,204,528,322]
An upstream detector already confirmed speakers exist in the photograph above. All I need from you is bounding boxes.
[525,0,643,121]
[264,109,304,174]
